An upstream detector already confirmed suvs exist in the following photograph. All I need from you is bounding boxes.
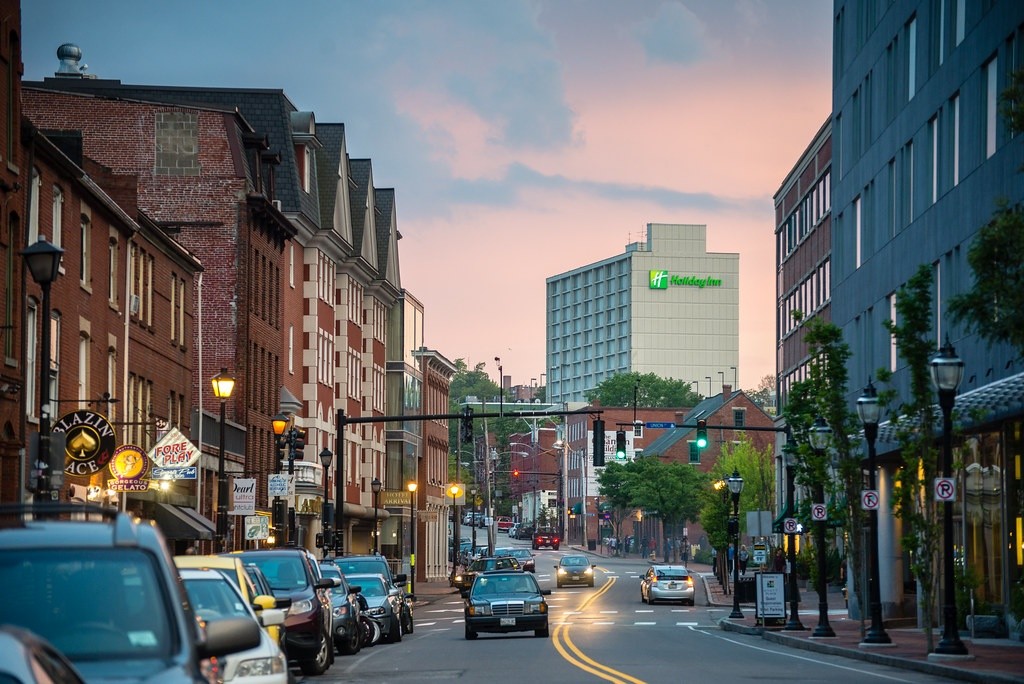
[0,504,414,684]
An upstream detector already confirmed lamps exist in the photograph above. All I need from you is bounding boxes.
[109,413,168,427]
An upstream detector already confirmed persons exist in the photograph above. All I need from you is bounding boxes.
[640,535,649,559]
[649,537,657,553]
[738,545,749,576]
[662,539,671,562]
[772,547,786,573]
[185,546,197,555]
[605,534,630,555]
[680,537,690,567]
[840,559,847,598]
[711,548,717,574]
[729,543,734,576]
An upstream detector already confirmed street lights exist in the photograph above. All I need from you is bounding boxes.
[455,437,477,487]
[782,435,805,630]
[271,412,291,547]
[211,367,234,553]
[18,234,65,501]
[561,364,569,402]
[856,375,891,644]
[692,381,698,396]
[928,332,970,655]
[319,447,333,559]
[540,374,546,386]
[550,368,556,406]
[705,377,711,397]
[371,478,381,554]
[531,378,536,398]
[731,367,736,393]
[470,486,477,556]
[727,465,743,618]
[509,442,537,530]
[483,397,529,556]
[407,479,418,601]
[450,482,458,569]
[552,439,587,547]
[718,372,724,387]
[807,415,836,636]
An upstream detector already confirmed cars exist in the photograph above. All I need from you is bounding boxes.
[553,556,596,588]
[449,509,560,550]
[639,565,695,606]
[449,537,551,640]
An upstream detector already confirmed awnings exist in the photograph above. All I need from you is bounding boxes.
[572,502,582,514]
[598,502,615,513]
[180,507,216,533]
[155,503,213,541]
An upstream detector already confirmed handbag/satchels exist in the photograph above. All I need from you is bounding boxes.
[746,551,749,562]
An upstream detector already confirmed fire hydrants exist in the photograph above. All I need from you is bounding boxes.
[650,551,655,561]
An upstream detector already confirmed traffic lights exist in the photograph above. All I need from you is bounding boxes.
[616,431,626,459]
[595,499,599,510]
[696,419,708,449]
[513,469,518,476]
[293,427,306,459]
